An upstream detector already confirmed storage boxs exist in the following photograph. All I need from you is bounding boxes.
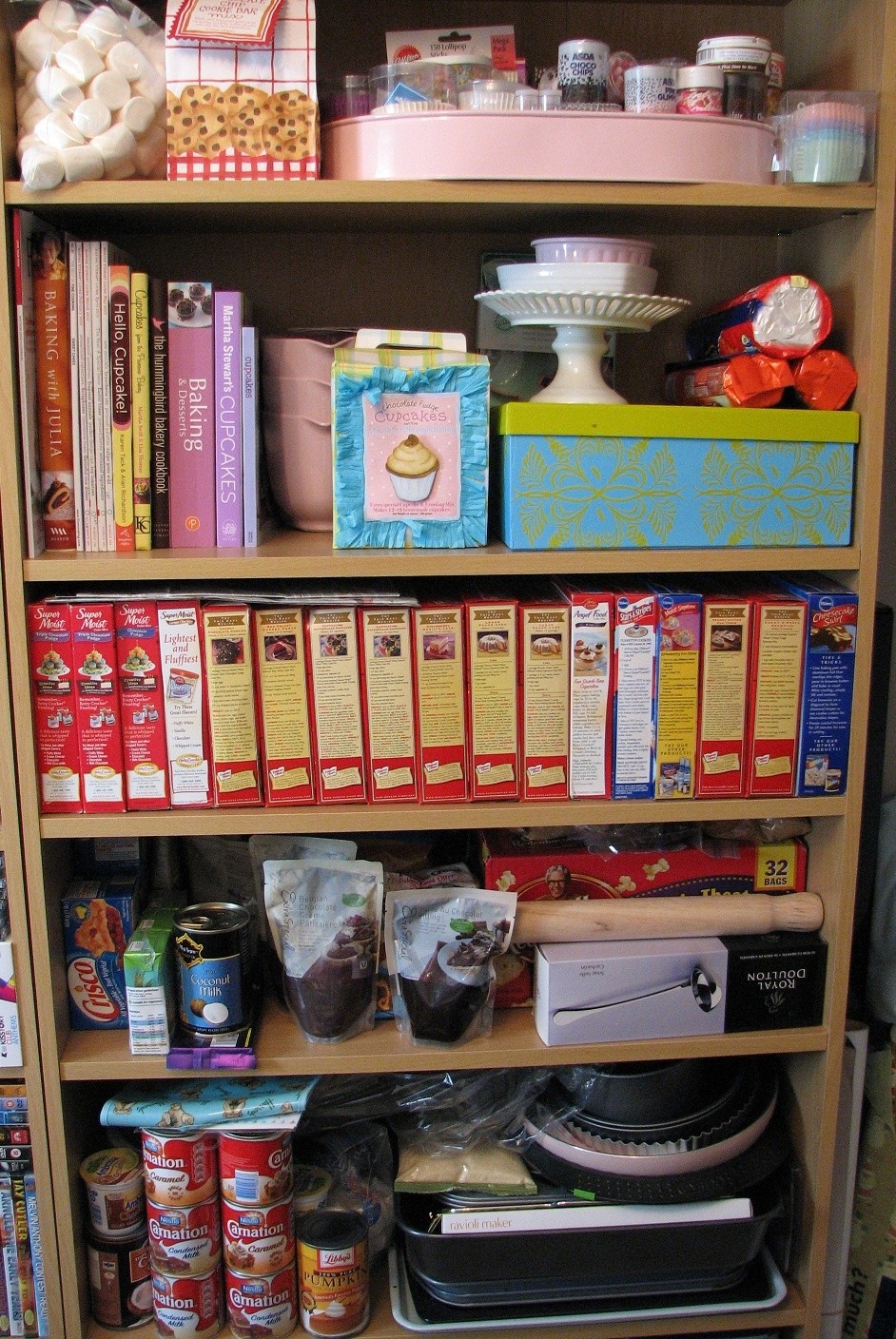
[534,931,829,1047]
[490,401,860,547]
[480,831,808,903]
[777,89,879,185]
[61,837,187,1055]
[0,933,24,1067]
[28,572,859,815]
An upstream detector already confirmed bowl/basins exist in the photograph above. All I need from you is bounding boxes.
[567,1058,704,1126]
[531,237,657,266]
[495,262,659,295]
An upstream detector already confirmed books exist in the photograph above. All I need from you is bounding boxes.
[10,206,262,560]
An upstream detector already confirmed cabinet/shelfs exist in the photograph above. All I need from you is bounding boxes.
[0,0,895,1339]
[0,555,66,1338]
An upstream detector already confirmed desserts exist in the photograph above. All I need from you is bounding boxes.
[573,639,604,663]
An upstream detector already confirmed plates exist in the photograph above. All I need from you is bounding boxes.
[543,1056,747,1144]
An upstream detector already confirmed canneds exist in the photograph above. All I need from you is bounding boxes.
[79,1125,371,1339]
[172,902,258,1038]
